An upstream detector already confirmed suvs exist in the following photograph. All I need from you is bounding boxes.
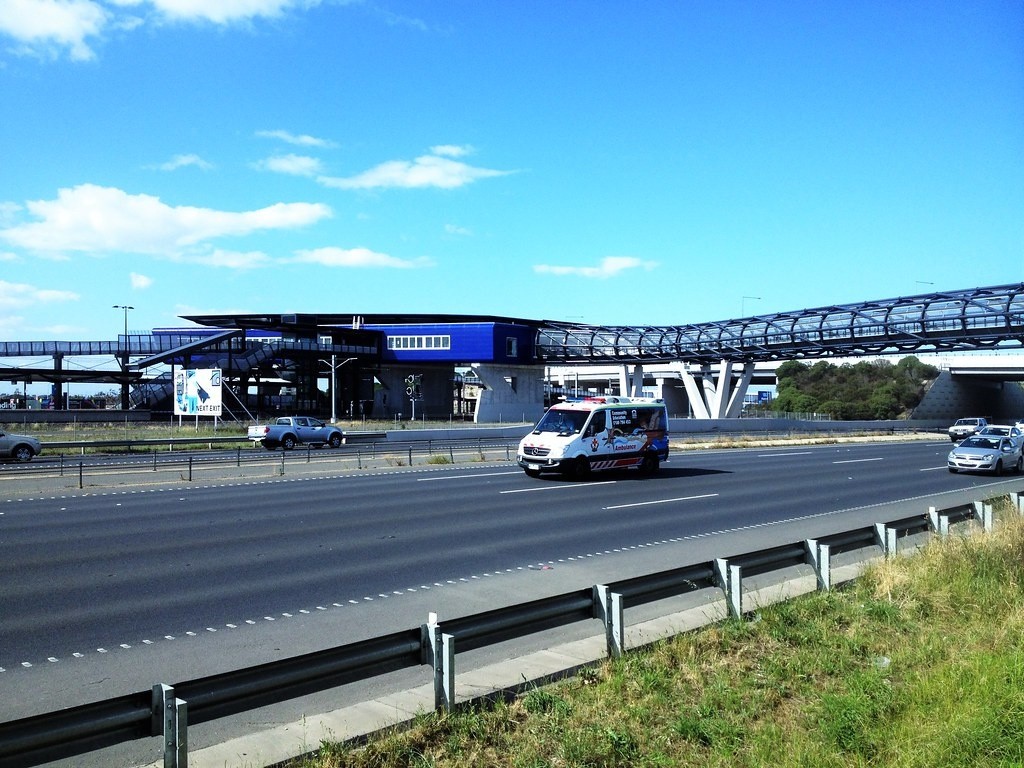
[948,418,987,442]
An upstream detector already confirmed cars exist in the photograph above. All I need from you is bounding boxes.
[1014,418,1024,434]
[975,424,1020,443]
[945,434,1024,476]
[0,429,43,462]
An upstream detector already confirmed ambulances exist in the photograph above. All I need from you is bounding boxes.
[516,393,671,480]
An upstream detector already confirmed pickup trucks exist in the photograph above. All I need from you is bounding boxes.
[246,416,344,452]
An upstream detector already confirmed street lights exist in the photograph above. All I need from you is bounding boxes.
[318,354,358,422]
[113,304,133,408]
